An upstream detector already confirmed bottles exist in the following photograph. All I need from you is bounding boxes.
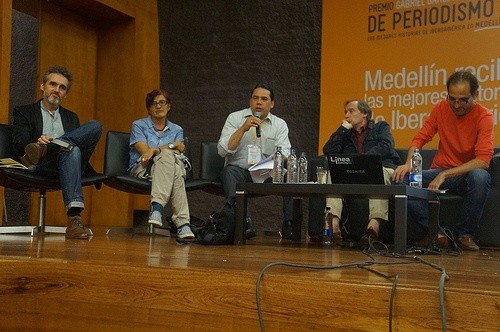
[410,149,423,189]
[322,207,333,247]
[273,146,284,183]
[298,152,307,183]
[287,148,298,183]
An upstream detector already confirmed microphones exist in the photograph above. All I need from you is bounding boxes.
[254,112,262,138]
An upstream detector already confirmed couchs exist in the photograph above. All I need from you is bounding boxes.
[300,148,500,251]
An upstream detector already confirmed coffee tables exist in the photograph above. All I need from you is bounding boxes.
[235,181,441,253]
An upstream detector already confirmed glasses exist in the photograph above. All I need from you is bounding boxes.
[150,100,168,107]
[446,95,473,105]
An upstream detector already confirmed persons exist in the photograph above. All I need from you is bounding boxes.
[390,71,494,251]
[129,89,194,238]
[11,65,103,238]
[217,85,294,239]
[323,99,403,240]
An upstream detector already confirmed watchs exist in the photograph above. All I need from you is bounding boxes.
[167,142,174,148]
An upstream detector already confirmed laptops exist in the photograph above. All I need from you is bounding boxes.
[326,153,386,184]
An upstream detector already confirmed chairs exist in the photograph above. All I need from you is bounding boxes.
[104,130,217,235]
[199,143,285,241]
[0,124,108,235]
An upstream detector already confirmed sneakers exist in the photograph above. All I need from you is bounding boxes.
[148,211,163,226]
[177,225,195,238]
[65,216,88,239]
[20,144,47,166]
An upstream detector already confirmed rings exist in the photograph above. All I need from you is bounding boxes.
[143,158,145,161]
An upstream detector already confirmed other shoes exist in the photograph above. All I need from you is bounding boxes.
[456,237,479,250]
[415,240,424,246]
[331,230,341,241]
[281,220,293,238]
[367,226,377,239]
[245,216,257,238]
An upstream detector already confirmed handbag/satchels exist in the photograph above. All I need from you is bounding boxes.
[198,201,235,244]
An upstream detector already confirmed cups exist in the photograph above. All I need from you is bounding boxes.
[317,169,327,184]
